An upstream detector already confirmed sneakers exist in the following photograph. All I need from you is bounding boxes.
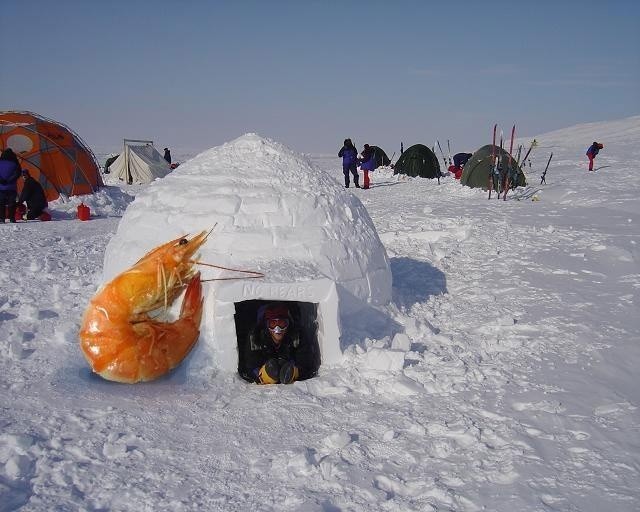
[345,185,369,189]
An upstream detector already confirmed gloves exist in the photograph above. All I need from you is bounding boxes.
[258,359,280,385]
[280,362,300,384]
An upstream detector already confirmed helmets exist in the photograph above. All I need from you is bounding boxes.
[263,304,292,319]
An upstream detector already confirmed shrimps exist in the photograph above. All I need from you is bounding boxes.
[79,222,264,382]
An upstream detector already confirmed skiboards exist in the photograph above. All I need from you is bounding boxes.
[488,123,536,201]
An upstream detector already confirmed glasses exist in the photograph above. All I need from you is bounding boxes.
[266,318,290,331]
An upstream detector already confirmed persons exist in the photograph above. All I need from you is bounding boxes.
[1,148,22,223]
[163,147,171,162]
[358,143,377,189]
[586,142,599,171]
[240,304,316,384]
[337,139,360,188]
[17,169,50,220]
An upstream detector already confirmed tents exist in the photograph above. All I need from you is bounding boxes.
[369,146,392,167]
[0,110,106,203]
[107,143,171,186]
[461,144,529,189]
[393,144,440,179]
